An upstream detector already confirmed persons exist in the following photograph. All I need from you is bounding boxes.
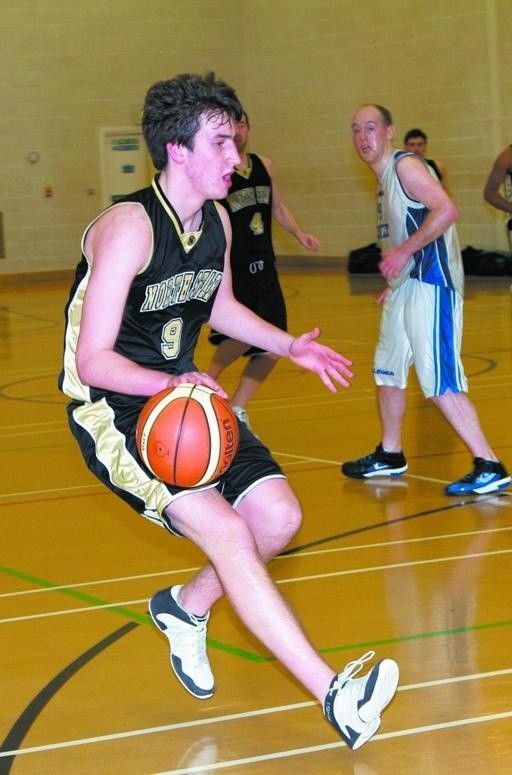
[342,105,512,496]
[404,127,451,199]
[56,69,401,750]
[204,111,321,412]
[482,144,512,250]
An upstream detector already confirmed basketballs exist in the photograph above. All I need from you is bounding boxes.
[135,385,237,485]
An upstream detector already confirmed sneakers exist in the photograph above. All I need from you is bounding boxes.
[230,405,260,444]
[321,656,400,751]
[148,582,216,700]
[444,455,512,495]
[342,441,409,480]
